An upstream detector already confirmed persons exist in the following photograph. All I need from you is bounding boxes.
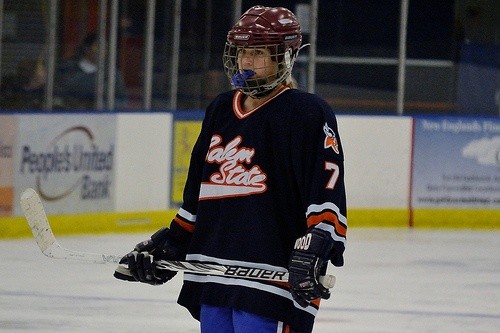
[113,6,348,333]
[18,26,130,112]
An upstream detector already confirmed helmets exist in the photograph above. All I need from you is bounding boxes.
[222,5,311,99]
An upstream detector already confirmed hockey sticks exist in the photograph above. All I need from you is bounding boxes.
[20,188,336,288]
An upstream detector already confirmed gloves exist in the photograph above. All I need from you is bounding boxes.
[287,228,345,308]
[114,227,186,286]
